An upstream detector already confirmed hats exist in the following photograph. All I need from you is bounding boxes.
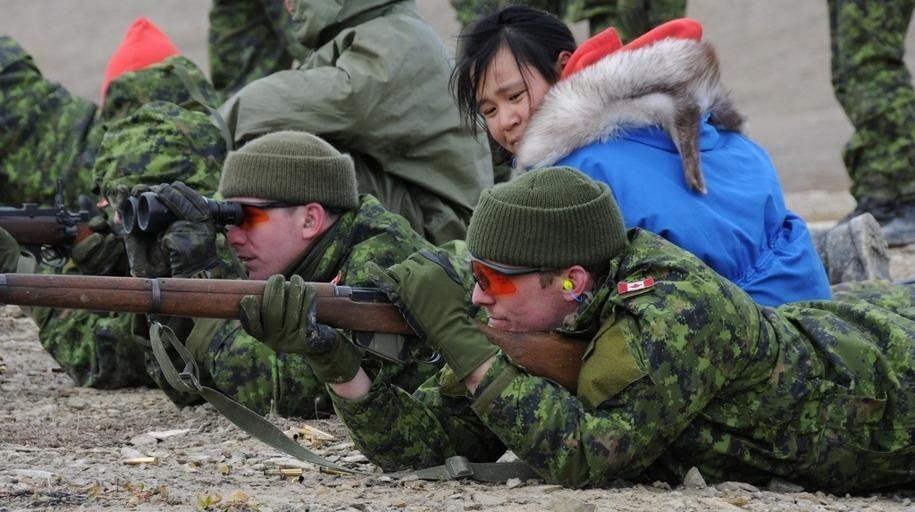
[220,130,359,208]
[467,165,625,266]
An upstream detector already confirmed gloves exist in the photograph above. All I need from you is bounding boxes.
[73,181,221,278]
[364,247,501,383]
[237,273,361,384]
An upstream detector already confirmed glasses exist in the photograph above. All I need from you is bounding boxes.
[469,255,555,296]
[239,200,293,230]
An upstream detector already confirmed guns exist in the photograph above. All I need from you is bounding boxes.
[1,272,590,396]
[0,178,94,268]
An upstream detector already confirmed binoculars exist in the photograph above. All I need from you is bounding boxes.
[122,191,244,235]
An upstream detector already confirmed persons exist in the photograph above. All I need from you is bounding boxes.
[0,0,915,501]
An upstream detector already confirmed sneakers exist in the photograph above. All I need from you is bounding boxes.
[835,193,915,247]
